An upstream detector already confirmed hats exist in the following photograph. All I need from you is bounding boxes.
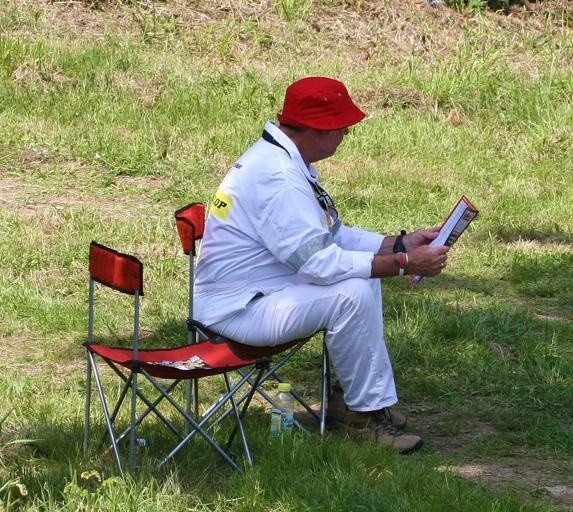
[275,75,366,131]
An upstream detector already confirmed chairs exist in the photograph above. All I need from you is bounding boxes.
[84,241,275,494]
[174,202,330,447]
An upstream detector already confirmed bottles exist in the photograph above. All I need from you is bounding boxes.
[270,384,295,445]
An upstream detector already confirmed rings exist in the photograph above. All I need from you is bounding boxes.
[439,262,444,270]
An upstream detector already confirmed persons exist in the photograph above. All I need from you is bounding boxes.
[190,73,451,454]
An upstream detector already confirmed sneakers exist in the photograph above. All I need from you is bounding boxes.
[340,407,424,455]
[326,381,406,431]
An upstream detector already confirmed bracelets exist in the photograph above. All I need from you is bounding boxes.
[395,250,407,280]
[394,228,408,252]
